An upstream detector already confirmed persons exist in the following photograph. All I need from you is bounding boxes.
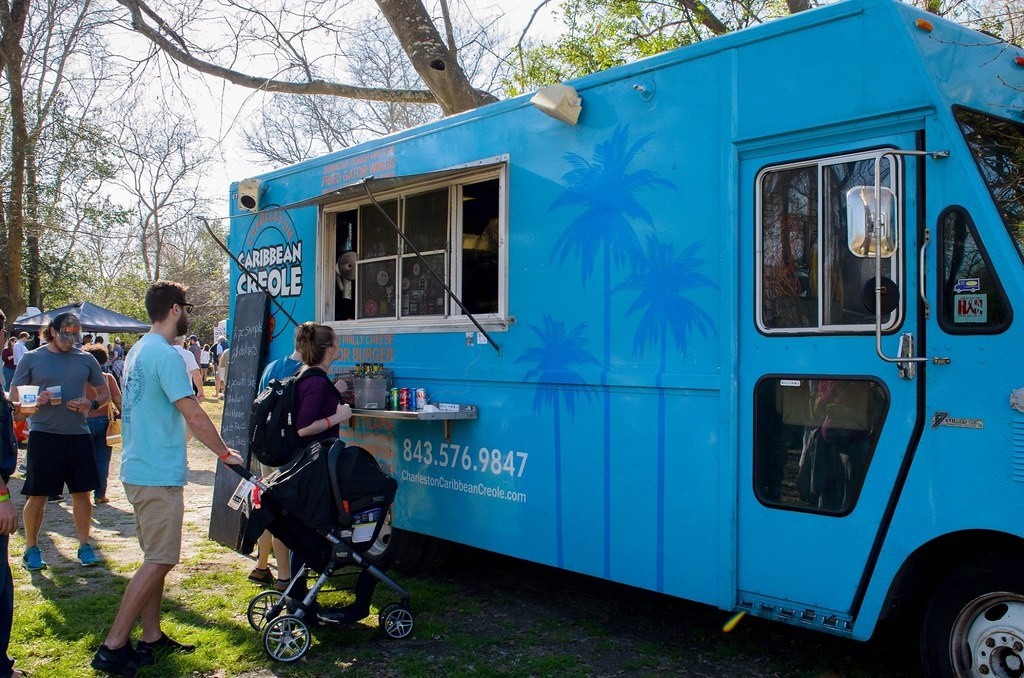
[247,325,348,591]
[91,279,244,678]
[284,323,352,628]
[797,377,886,513]
[3,330,230,505]
[0,308,27,678]
[8,313,111,572]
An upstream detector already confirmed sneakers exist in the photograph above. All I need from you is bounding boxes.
[48,496,66,503]
[247,568,276,585]
[271,578,290,594]
[78,543,100,566]
[91,640,159,671]
[22,546,46,570]
[136,631,195,665]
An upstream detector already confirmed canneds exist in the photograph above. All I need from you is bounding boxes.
[389,387,427,409]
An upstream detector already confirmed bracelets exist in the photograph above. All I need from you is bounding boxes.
[325,417,331,429]
[219,450,230,460]
[0,488,9,496]
[0,493,10,502]
[90,400,99,411]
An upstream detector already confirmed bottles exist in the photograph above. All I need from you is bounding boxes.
[353,361,386,380]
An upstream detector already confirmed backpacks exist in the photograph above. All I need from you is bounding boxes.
[249,367,328,466]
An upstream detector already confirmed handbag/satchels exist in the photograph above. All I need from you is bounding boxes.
[216,343,223,354]
[106,373,123,447]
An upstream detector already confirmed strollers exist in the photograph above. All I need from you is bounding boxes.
[225,438,417,664]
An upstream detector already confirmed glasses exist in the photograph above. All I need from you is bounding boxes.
[3,330,8,338]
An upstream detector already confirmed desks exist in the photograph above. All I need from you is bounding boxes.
[348,408,476,441]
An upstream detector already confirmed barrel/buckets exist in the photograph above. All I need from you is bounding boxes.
[352,378,388,410]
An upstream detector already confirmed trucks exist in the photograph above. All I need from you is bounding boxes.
[226,0,1024,678]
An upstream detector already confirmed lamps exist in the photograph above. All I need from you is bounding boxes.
[530,84,584,126]
[236,178,264,211]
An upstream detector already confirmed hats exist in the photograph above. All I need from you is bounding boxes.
[138,335,143,340]
[115,337,122,344]
[187,335,198,339]
[53,313,82,344]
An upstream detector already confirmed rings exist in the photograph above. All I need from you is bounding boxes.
[77,408,79,411]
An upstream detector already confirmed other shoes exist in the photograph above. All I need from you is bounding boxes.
[95,497,109,504]
[218,395,225,399]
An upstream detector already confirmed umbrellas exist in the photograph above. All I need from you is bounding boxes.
[11,302,152,332]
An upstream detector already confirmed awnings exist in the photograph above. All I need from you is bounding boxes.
[203,160,505,221]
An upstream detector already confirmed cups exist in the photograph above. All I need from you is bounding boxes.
[16,385,40,414]
[46,385,62,406]
